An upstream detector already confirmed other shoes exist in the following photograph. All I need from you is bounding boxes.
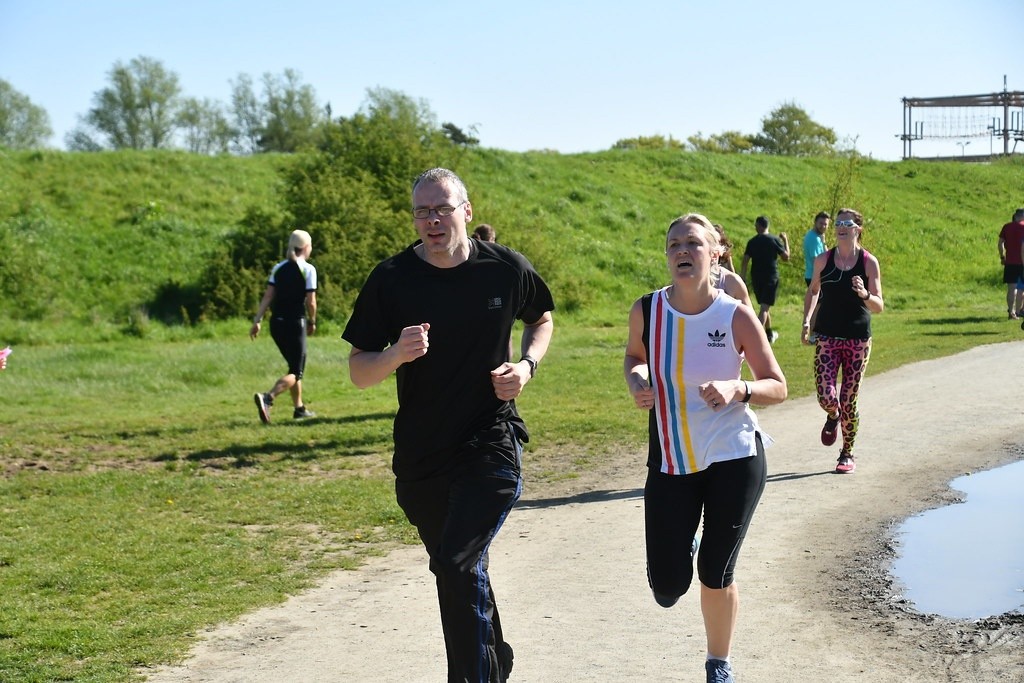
[1009,313,1019,319]
[1016,311,1024,317]
[769,331,779,343]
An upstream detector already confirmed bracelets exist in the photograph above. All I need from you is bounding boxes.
[310,322,316,325]
[1001,256,1005,259]
[254,317,262,323]
[740,379,752,403]
[823,242,827,245]
[862,289,870,300]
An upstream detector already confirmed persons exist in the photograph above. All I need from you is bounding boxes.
[801,208,884,473]
[623,213,788,683]
[0,351,7,370]
[740,216,791,344]
[341,167,555,683]
[248,230,318,422]
[472,224,496,243]
[998,208,1024,331]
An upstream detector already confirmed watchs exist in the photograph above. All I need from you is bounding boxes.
[520,355,537,378]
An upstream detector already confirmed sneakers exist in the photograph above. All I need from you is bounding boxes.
[821,407,841,446]
[705,659,732,683]
[690,538,698,553]
[836,448,855,474]
[294,409,316,420]
[255,393,273,424]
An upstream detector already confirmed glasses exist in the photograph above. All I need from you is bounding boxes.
[411,201,466,219]
[835,219,859,229]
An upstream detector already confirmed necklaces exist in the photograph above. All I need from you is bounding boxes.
[838,249,856,270]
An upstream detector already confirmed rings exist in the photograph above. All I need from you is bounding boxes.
[644,401,646,406]
[712,400,717,406]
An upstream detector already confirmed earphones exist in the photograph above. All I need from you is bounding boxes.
[855,231,858,236]
